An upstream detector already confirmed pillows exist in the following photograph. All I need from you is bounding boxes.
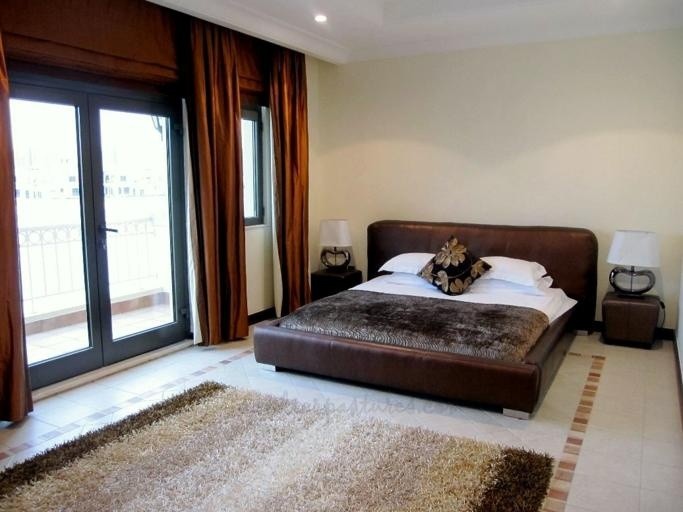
[385,272,436,290]
[416,234,491,296]
[376,251,434,275]
[469,275,553,297]
[477,255,547,288]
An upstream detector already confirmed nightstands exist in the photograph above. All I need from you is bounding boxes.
[599,291,661,352]
[310,268,362,302]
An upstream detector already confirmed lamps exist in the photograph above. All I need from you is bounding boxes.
[318,219,353,271]
[604,229,660,298]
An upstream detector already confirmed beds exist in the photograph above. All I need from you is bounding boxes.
[252,218,599,421]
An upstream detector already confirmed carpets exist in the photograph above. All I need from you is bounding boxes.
[1,380,555,512]
